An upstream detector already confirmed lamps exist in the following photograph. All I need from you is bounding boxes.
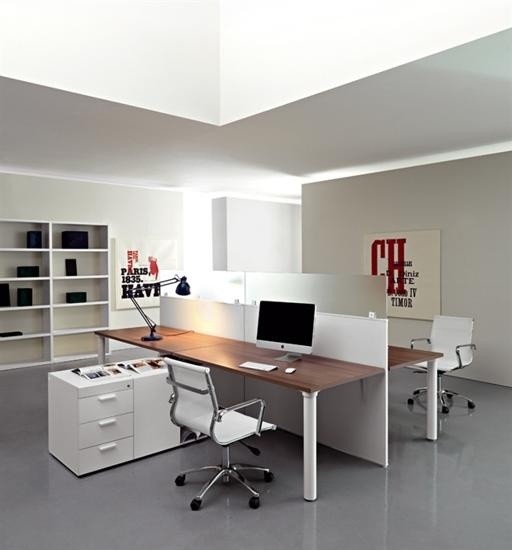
[125,275,191,342]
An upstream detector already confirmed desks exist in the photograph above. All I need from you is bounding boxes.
[94,270,444,502]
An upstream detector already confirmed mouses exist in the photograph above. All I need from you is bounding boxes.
[285,367,296,373]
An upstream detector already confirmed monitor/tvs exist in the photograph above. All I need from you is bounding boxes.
[256,300,316,363]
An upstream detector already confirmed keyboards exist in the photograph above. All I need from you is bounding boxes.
[239,361,278,373]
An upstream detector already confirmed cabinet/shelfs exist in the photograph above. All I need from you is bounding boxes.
[0,218,111,370]
[48,363,134,477]
[114,353,209,460]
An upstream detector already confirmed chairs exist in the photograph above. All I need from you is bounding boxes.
[408,315,477,413]
[163,355,277,510]
